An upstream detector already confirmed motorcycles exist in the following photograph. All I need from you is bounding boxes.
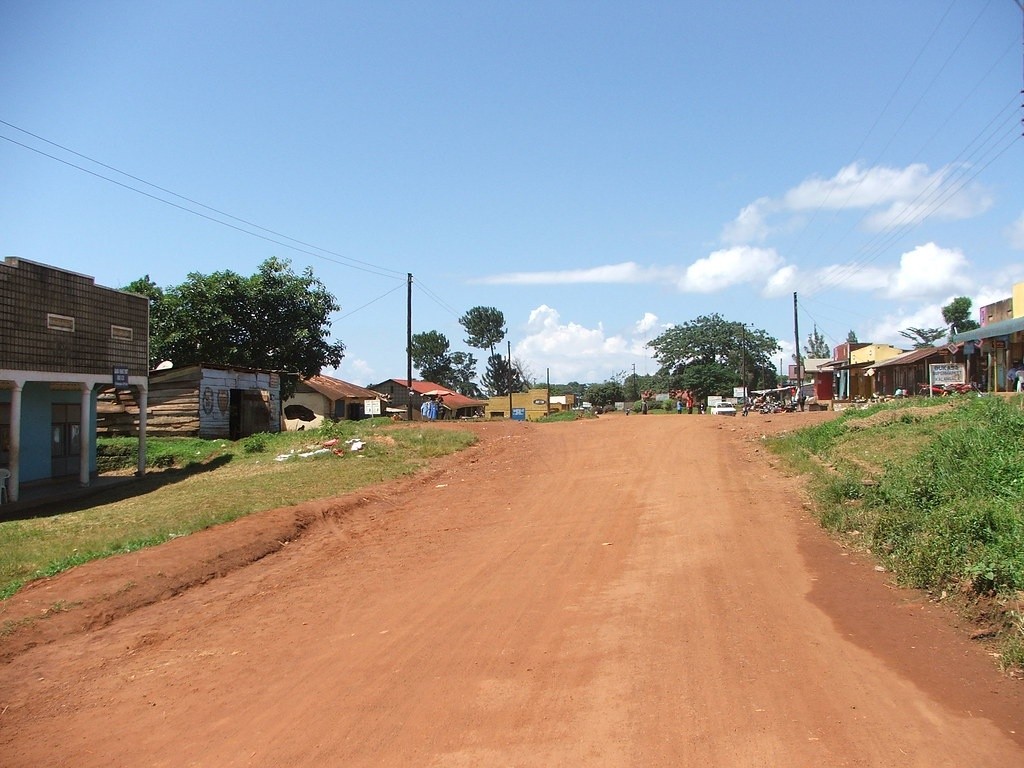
[741,406,751,416]
[916,376,973,398]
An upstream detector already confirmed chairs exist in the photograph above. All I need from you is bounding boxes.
[0,469,11,504]
[1015,371,1024,393]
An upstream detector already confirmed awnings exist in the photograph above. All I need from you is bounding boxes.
[953,316,1024,343]
[834,361,875,375]
[861,348,937,370]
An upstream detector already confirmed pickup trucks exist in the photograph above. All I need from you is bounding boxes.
[711,402,736,417]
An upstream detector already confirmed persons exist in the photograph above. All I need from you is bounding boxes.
[626,408,630,415]
[677,398,683,414]
[686,397,693,414]
[697,403,704,414]
[642,397,648,414]
[1006,365,1024,392]
[480,406,485,417]
[895,387,909,398]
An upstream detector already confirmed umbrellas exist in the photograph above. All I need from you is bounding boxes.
[420,388,456,398]
